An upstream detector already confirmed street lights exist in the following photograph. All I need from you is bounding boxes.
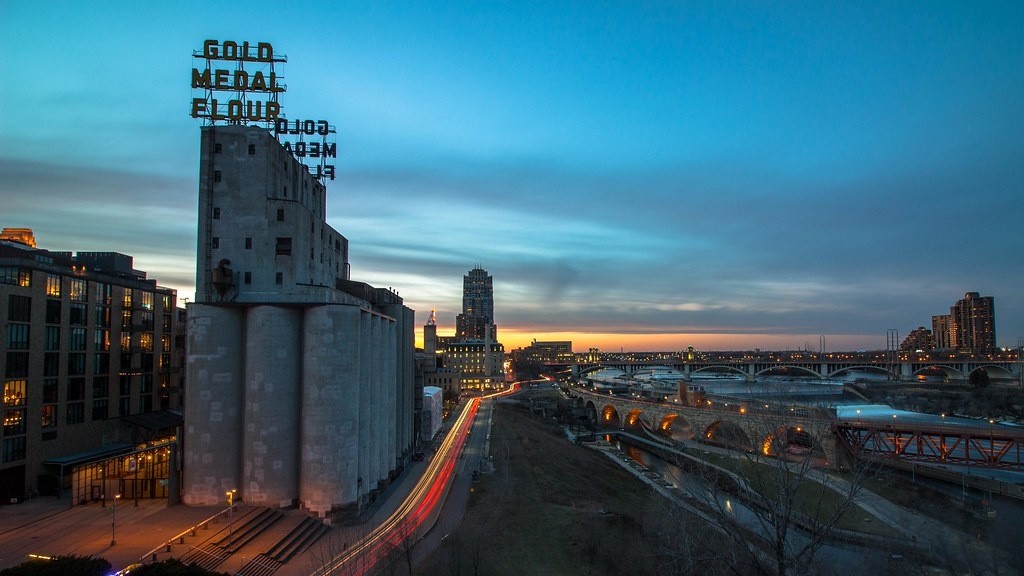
[226,491,233,548]
[110,493,121,545]
